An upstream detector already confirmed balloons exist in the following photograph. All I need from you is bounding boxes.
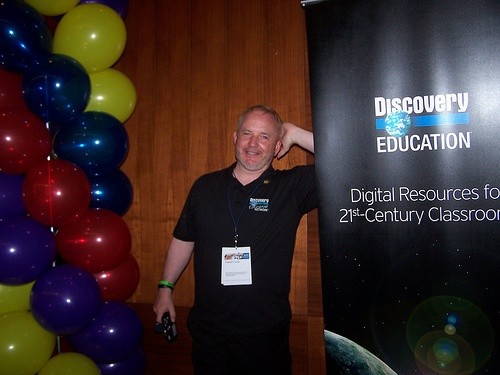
[0,0,128,74]
[23,159,92,227]
[0,172,27,218]
[0,280,35,311]
[0,311,56,375]
[0,218,57,286]
[51,4,137,124]
[68,301,143,363]
[55,208,132,273]
[36,354,101,375]
[53,111,134,216]
[29,265,101,336]
[22,54,91,132]
[91,253,140,301]
[0,69,52,174]
[99,348,146,375]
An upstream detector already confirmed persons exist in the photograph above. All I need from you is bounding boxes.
[152,104,317,375]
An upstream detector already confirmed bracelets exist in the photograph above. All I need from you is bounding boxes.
[158,284,174,288]
[159,280,174,287]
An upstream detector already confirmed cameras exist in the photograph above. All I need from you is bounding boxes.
[154,312,178,342]
